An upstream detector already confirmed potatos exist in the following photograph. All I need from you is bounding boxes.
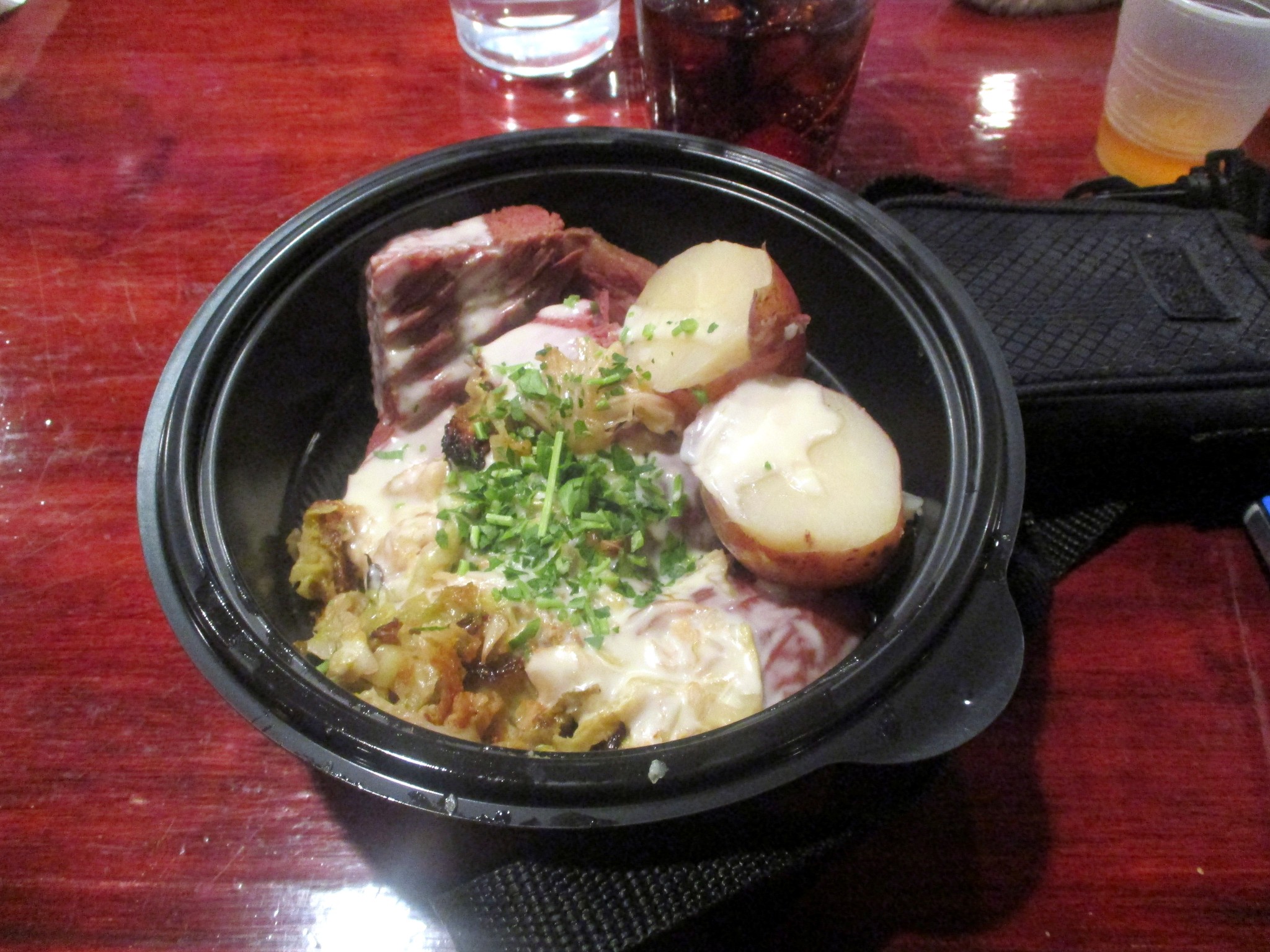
[620,238,809,406]
[689,374,906,588]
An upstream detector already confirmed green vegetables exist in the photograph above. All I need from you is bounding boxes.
[373,292,770,655]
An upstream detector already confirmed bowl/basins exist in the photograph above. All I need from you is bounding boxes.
[135,125,1025,827]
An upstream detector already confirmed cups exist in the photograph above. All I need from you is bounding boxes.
[633,0,875,176]
[449,0,620,76]
[1096,1,1270,189]
